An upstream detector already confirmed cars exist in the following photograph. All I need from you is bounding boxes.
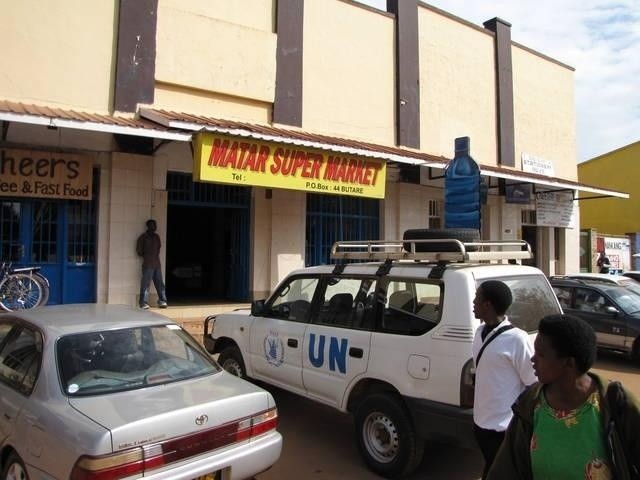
[0,303,284,480]
[560,273,640,297]
[621,272,640,284]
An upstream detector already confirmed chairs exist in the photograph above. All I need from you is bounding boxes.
[360,292,375,327]
[319,293,353,323]
[93,332,145,373]
[383,290,414,333]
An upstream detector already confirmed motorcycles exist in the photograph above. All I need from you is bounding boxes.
[0,260,51,312]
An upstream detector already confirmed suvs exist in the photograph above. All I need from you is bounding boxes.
[547,274,640,364]
[204,228,566,477]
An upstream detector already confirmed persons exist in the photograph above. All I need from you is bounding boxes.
[135,219,169,309]
[471,279,540,479]
[486,314,639,479]
[596,251,612,275]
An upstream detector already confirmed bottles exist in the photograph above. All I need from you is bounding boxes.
[444,136,481,230]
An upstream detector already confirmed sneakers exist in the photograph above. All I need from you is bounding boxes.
[159,301,167,308]
[142,304,150,309]
